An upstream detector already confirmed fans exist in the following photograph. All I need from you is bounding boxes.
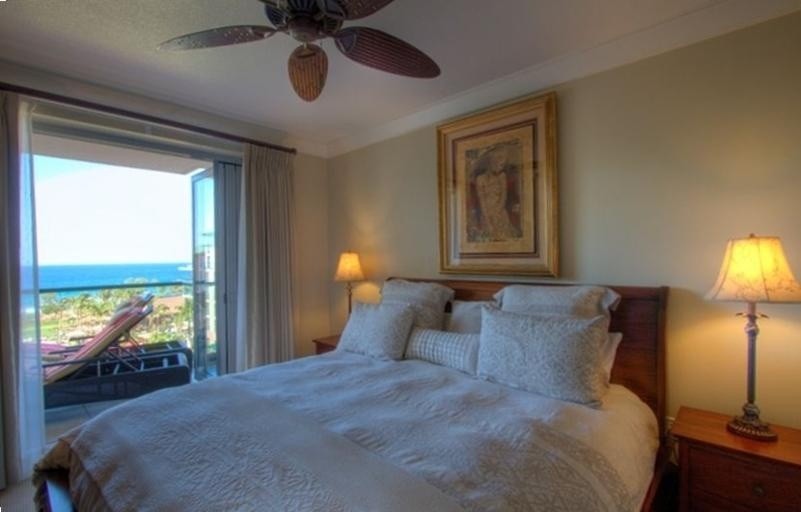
[158,1,441,102]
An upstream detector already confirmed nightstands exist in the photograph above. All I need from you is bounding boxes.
[669,404,801,512]
[312,335,340,354]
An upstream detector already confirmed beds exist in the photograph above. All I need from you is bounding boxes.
[32,274,671,511]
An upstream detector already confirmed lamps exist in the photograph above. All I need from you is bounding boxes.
[704,233,801,443]
[333,250,366,315]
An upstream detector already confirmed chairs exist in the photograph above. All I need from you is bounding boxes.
[44,292,192,410]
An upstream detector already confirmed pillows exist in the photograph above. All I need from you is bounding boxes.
[335,278,625,409]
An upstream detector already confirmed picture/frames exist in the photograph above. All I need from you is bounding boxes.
[436,91,559,276]
